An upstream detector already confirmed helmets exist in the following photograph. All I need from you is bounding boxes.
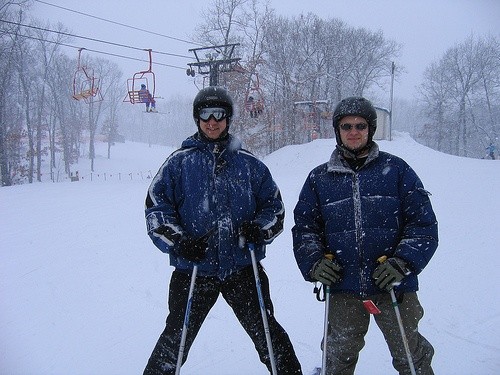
[193,87,233,134]
[332,96,377,146]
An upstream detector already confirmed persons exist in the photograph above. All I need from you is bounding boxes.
[291,97,439,375]
[138,84,158,112]
[485,142,495,160]
[247,96,258,118]
[142,86,303,375]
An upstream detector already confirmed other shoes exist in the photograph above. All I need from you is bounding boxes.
[146,107,148,111]
[150,108,155,111]
[251,116,253,118]
[254,116,258,118]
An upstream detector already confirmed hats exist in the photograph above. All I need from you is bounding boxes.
[249,96,254,99]
[141,84,146,88]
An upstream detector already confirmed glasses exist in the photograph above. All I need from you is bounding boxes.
[197,107,228,122]
[339,123,369,131]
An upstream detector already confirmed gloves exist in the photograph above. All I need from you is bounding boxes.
[373,258,411,290]
[236,223,264,255]
[182,241,208,264]
[312,258,340,285]
